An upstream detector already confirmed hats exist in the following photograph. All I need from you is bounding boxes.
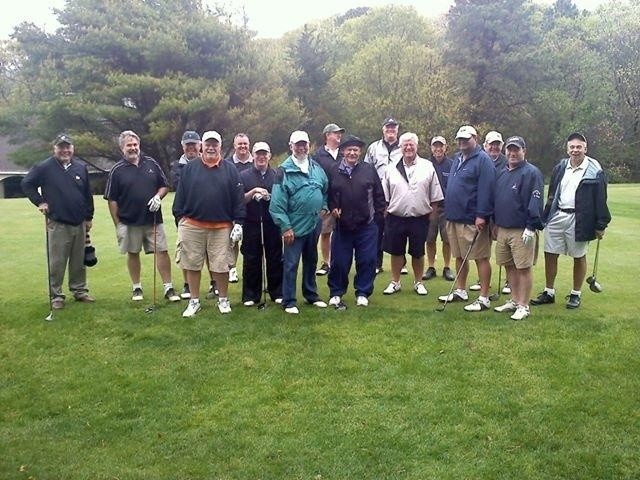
[486,131,503,144]
[253,142,271,152]
[568,133,587,142]
[289,130,309,143]
[323,124,345,134]
[54,134,71,145]
[505,136,525,149]
[430,136,446,145]
[182,131,199,144]
[202,131,220,142]
[382,117,398,127]
[456,126,476,139]
[337,135,365,147]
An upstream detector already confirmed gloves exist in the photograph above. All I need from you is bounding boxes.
[230,224,242,241]
[522,229,535,245]
[148,195,160,212]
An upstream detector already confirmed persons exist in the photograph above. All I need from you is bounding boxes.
[493,135,545,321]
[529,131,613,309]
[223,132,256,283]
[422,135,457,281]
[168,131,249,318]
[436,125,495,312]
[169,131,217,301]
[380,131,447,295]
[326,134,388,307]
[470,130,515,296]
[102,129,182,302]
[314,123,346,278]
[363,116,411,277]
[240,140,285,308]
[21,133,96,310]
[267,129,331,314]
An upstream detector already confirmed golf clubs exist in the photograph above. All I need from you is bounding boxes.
[145,211,157,313]
[489,266,501,301]
[433,228,476,312]
[258,199,268,310]
[45,212,54,321]
[586,237,603,292]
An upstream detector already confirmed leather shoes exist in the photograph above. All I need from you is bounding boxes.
[422,268,436,279]
[53,302,64,308]
[442,267,455,281]
[81,296,95,302]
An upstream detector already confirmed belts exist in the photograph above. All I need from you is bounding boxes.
[558,206,576,212]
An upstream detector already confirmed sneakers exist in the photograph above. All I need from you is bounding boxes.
[132,288,143,301]
[464,298,490,311]
[438,290,468,302]
[511,305,530,320]
[400,266,406,273]
[502,281,511,293]
[529,291,555,306]
[470,280,491,290]
[383,280,401,293]
[414,281,427,294]
[207,285,216,299]
[356,295,368,306]
[162,282,202,318]
[274,299,299,313]
[493,299,519,312]
[304,298,326,309]
[316,260,329,274]
[565,294,581,308]
[244,300,254,306]
[228,266,238,282]
[216,300,231,314]
[330,296,340,305]
